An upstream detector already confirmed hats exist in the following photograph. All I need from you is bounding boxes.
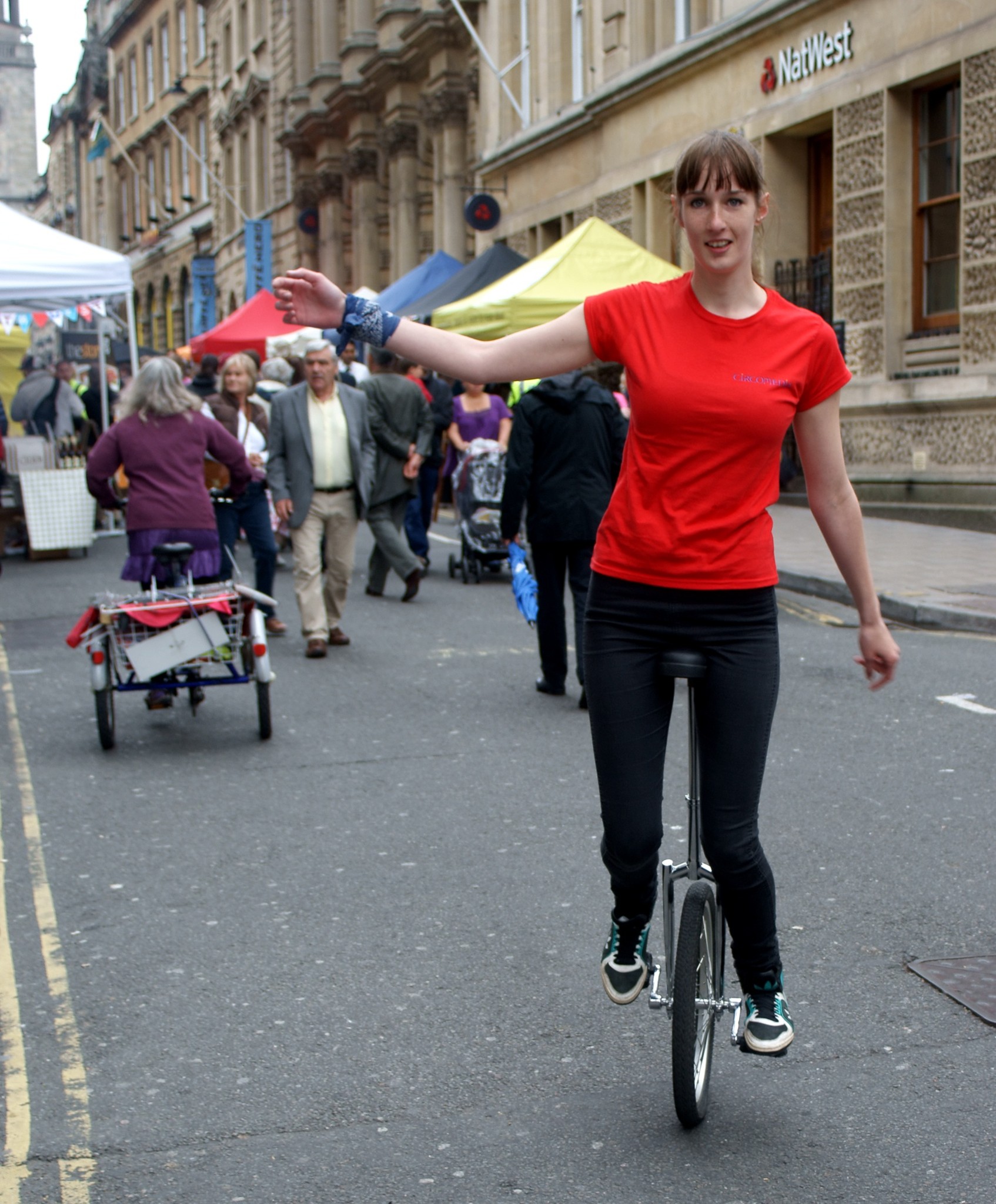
[19,356,34,369]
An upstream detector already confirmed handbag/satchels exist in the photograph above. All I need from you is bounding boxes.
[204,458,230,492]
[29,397,56,433]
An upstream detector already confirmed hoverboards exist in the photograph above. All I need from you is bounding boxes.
[635,631,787,1133]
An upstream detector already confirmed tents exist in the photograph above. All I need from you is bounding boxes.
[0,202,142,538]
[169,216,695,408]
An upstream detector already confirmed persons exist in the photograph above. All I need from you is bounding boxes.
[271,131,902,1055]
[87,342,629,712]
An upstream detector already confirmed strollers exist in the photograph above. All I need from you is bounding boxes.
[446,439,528,582]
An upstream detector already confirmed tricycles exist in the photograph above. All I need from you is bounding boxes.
[66,487,280,749]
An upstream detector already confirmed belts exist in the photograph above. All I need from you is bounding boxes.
[314,485,351,494]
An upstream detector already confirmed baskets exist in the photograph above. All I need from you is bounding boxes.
[104,582,243,673]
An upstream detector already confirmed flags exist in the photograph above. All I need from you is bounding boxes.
[88,119,108,163]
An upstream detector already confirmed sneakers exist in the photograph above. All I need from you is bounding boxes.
[599,907,650,1005]
[739,968,794,1053]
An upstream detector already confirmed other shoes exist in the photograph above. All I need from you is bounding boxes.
[578,690,588,709]
[535,676,566,695]
[188,691,204,703]
[144,690,167,707]
[489,560,501,573]
[305,639,327,657]
[365,587,381,597]
[330,627,350,645]
[401,568,424,601]
[266,618,287,633]
